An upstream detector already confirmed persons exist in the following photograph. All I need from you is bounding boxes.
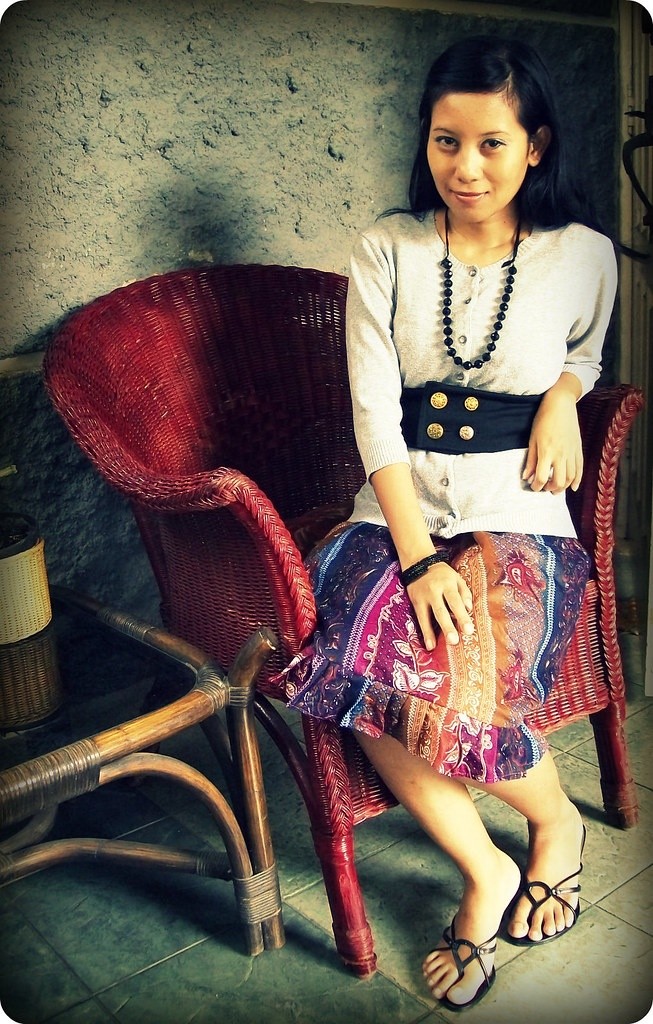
[284,37,619,1014]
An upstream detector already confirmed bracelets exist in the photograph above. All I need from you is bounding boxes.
[401,553,445,586]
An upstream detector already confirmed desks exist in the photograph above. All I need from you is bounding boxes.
[0,586,287,958]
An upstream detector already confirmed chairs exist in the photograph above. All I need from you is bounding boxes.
[40,264,647,982]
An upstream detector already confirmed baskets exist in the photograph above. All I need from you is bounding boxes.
[0,618,65,731]
[0,512,54,645]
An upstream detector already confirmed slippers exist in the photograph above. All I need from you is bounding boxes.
[422,859,525,1012]
[507,823,586,946]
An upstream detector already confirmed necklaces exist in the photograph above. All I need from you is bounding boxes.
[441,208,523,371]
